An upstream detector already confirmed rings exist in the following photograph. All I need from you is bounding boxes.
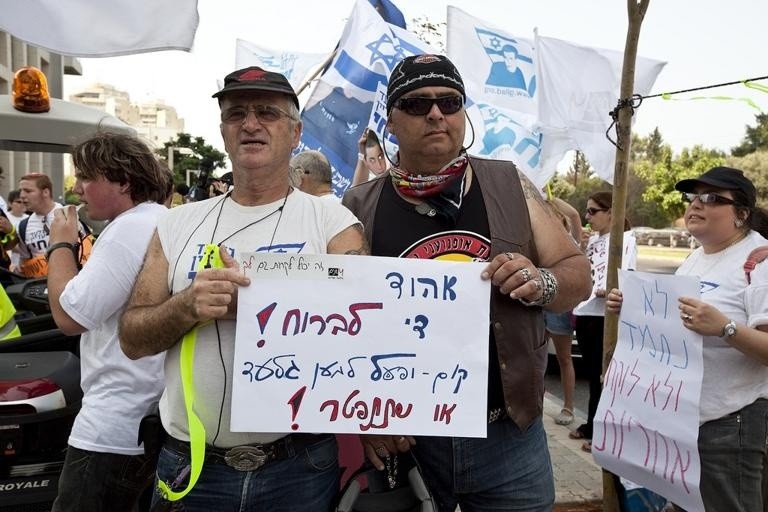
[684,314,693,321]
[396,434,407,444]
[373,445,387,453]
[505,251,513,260]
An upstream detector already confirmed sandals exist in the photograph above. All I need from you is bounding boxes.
[555,407,576,425]
[569,431,583,439]
[582,441,592,452]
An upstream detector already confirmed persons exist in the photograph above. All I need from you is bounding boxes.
[341,52,593,512]
[287,150,340,205]
[45,131,180,511]
[0,162,246,285]
[350,126,372,190]
[604,166,767,511]
[535,188,583,427]
[362,135,386,176]
[115,67,370,511]
[486,44,527,91]
[569,191,636,453]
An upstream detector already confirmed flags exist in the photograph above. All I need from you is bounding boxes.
[291,0,444,199]
[530,34,668,187]
[236,38,301,83]
[443,5,558,192]
[0,0,202,57]
[364,80,401,181]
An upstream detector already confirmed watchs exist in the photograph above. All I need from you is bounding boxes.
[719,319,737,340]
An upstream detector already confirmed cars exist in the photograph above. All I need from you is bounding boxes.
[630,230,697,272]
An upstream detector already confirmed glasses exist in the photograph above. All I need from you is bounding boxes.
[585,207,605,216]
[391,95,463,116]
[680,191,741,206]
[220,106,298,125]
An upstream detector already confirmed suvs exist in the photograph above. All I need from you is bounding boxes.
[1,67,139,507]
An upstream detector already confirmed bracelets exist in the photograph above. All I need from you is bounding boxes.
[357,151,366,162]
[518,267,558,306]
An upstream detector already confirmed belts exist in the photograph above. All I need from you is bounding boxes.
[163,434,331,474]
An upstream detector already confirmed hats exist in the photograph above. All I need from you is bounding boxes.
[212,66,299,110]
[675,167,757,211]
[386,55,466,118]
